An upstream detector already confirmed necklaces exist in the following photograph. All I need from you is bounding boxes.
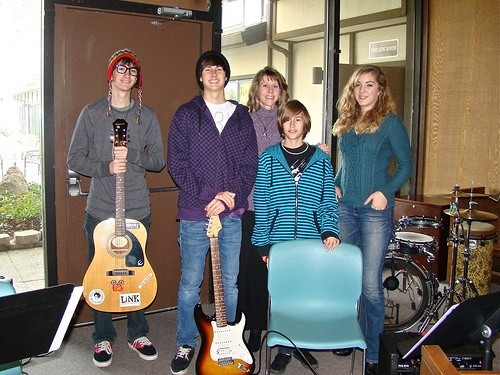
[112,99,132,121]
[255,112,277,138]
[281,139,309,155]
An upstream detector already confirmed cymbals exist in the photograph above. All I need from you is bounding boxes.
[444,208,498,221]
[440,193,490,199]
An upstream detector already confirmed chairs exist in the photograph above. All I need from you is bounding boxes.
[265,239,367,375]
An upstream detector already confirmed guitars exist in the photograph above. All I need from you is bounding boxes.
[195,216,256,375]
[82,118,158,313]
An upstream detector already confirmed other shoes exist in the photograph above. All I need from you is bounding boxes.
[333,347,354,356]
[269,352,292,374]
[366,362,379,375]
[292,350,320,370]
[248,328,262,352]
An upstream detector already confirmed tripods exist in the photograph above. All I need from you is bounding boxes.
[418,185,481,336]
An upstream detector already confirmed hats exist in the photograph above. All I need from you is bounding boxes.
[107,48,142,125]
[195,50,231,90]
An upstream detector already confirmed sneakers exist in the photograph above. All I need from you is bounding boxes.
[93,340,113,368]
[127,336,158,361]
[169,345,196,375]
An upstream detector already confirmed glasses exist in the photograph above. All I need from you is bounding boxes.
[115,65,139,76]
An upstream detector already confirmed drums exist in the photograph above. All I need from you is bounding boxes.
[377,215,496,332]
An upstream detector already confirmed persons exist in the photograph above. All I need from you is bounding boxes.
[158,51,413,375]
[67,49,166,367]
